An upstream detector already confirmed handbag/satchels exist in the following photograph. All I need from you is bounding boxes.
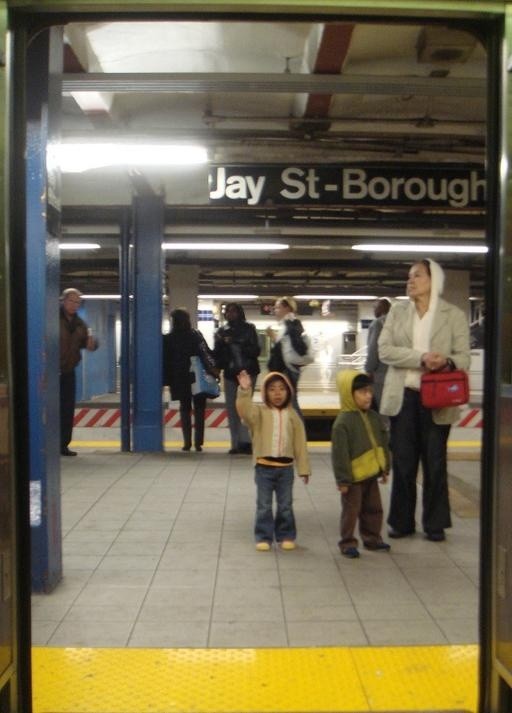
[189,356,219,398]
[350,446,387,482]
[421,368,470,408]
[282,332,315,366]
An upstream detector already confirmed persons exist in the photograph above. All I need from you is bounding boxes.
[265,296,307,422]
[212,302,261,454]
[236,370,313,551]
[330,371,391,557]
[377,258,471,541]
[59,289,100,456]
[366,298,393,475]
[161,306,222,452]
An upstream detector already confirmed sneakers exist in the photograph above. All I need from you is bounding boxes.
[370,543,390,550]
[428,531,446,540]
[255,541,271,551]
[387,529,409,538]
[340,547,360,558]
[280,540,295,549]
